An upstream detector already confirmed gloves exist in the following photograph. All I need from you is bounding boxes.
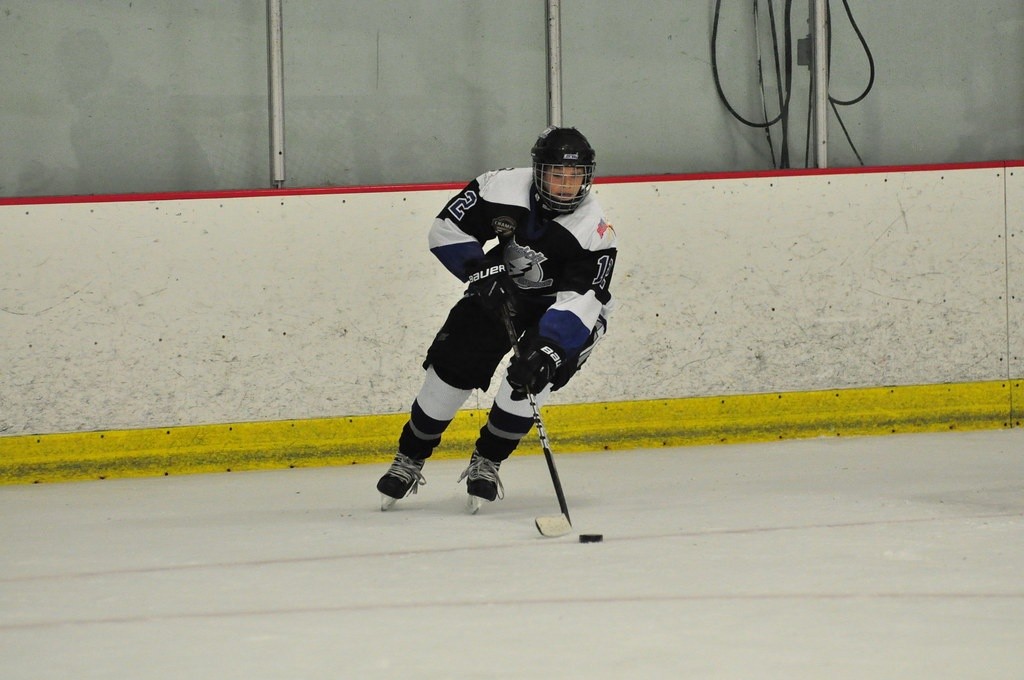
[507,335,565,401]
[463,258,525,318]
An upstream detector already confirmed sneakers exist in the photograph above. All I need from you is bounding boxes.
[376,449,425,511]
[457,447,505,515]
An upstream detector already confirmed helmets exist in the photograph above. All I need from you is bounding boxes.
[531,125,596,215]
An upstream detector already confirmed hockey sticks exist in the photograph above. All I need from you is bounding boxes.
[495,289,576,539]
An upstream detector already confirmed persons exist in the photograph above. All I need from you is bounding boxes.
[376,125,618,514]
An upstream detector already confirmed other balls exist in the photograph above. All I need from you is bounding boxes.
[578,533,604,544]
